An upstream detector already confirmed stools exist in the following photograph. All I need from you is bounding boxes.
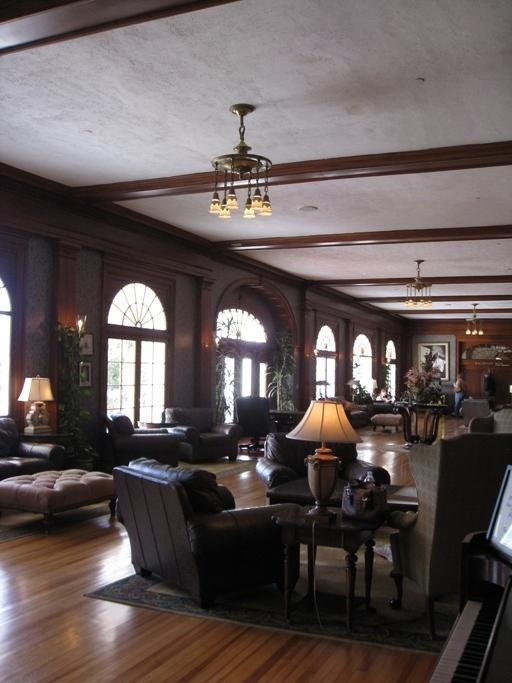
[370,413,404,432]
[0,469,119,534]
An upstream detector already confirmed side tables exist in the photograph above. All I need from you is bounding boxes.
[271,504,386,633]
[267,474,418,520]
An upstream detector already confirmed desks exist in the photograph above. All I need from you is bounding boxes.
[269,410,305,433]
[19,432,74,468]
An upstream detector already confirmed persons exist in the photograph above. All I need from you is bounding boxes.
[450,373,465,415]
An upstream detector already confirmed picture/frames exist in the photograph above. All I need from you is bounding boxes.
[78,332,96,357]
[416,341,450,382]
[78,361,94,388]
[484,465,512,566]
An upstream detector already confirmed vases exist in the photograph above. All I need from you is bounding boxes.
[73,457,94,471]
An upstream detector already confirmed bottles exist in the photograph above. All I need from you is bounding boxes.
[362,470,376,483]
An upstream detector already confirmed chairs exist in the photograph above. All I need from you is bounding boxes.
[104,397,277,465]
[390,398,512,642]
[0,417,68,481]
[319,393,393,428]
[113,457,301,609]
[255,432,390,506]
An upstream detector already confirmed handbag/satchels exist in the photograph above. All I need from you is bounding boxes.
[456,387,462,393]
[341,478,385,525]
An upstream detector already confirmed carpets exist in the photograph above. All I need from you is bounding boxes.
[176,459,258,480]
[82,528,459,655]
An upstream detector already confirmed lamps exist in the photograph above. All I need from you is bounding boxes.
[405,260,433,308]
[465,304,484,336]
[208,104,273,219]
[17,374,54,434]
[285,399,363,527]
